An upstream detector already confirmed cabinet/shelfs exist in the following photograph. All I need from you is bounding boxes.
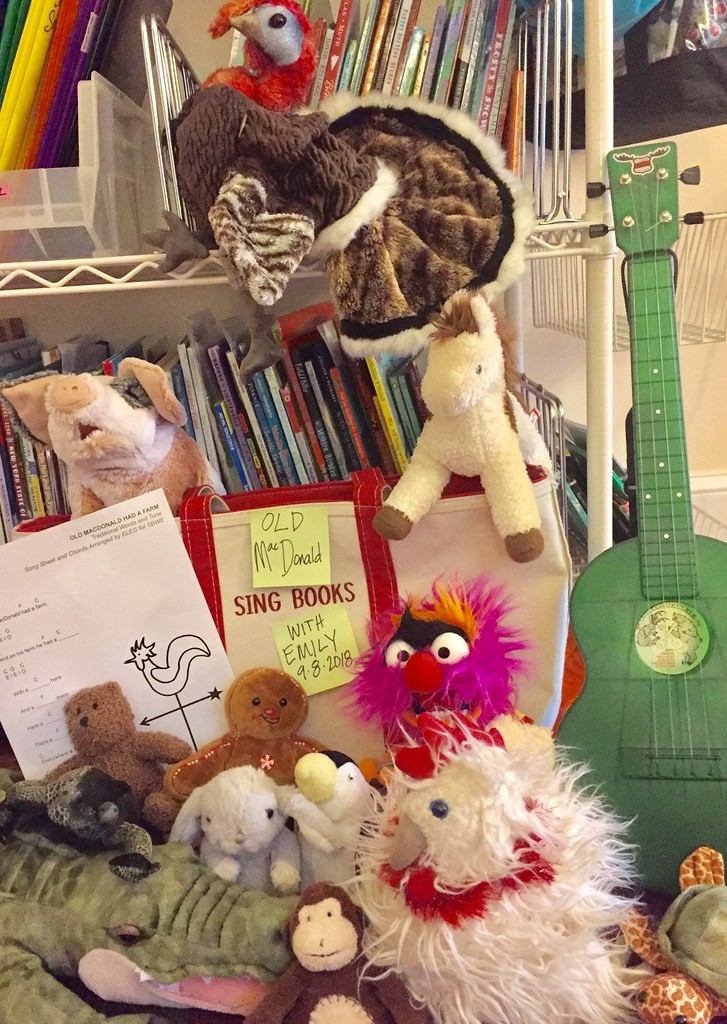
[0,0,622,561]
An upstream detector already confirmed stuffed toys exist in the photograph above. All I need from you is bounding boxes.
[0,86,727,1024]
[205,0,321,115]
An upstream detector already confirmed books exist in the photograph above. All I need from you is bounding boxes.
[0,0,520,546]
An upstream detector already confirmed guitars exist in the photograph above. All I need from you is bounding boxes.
[551,140,726,899]
